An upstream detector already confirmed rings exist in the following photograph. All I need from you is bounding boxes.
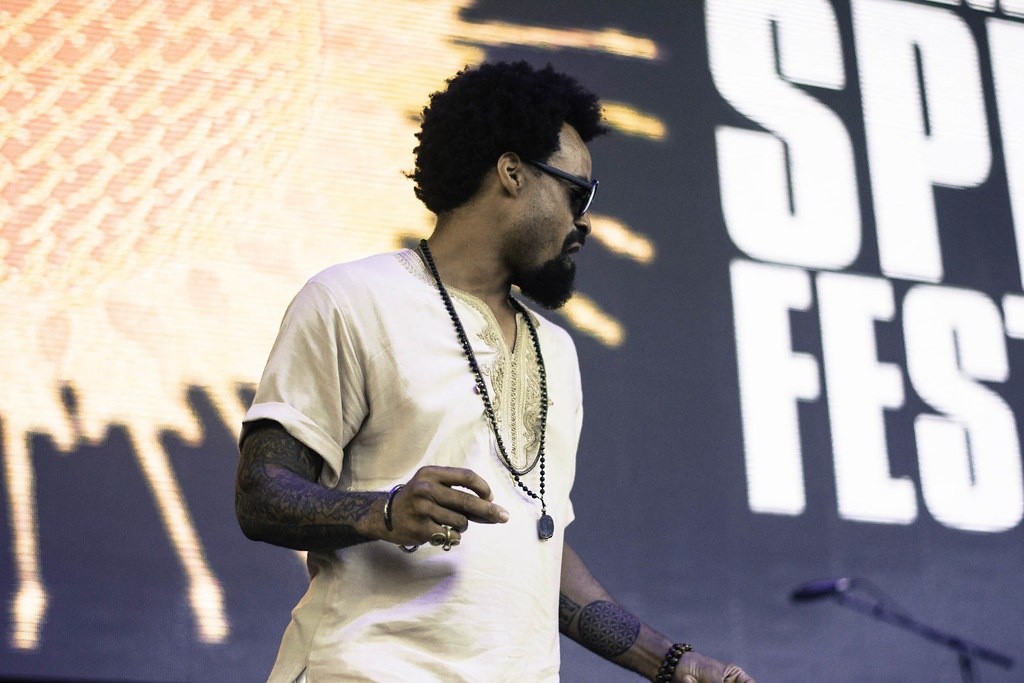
[429,526,459,551]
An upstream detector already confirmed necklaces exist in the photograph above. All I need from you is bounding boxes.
[419,239,555,540]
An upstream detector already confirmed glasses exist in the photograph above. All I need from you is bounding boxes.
[526,154,598,217]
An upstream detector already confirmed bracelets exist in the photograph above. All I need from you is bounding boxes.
[656,642,694,683]
[383,484,420,553]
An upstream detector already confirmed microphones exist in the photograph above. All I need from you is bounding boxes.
[792,577,856,604]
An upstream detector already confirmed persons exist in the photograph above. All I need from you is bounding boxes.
[235,62,755,683]
